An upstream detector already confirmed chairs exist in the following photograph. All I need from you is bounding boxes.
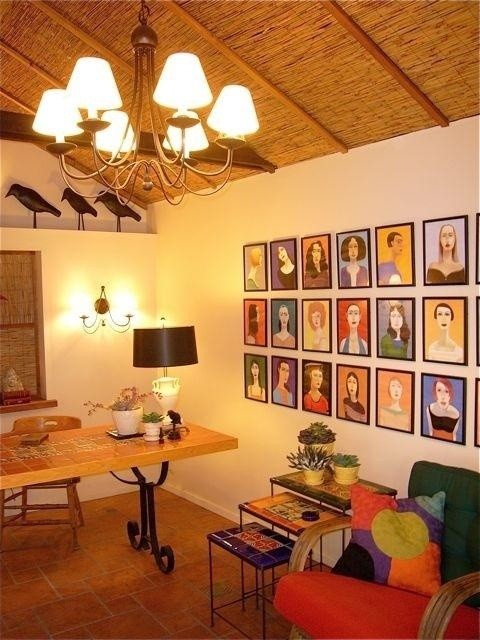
[0,416,84,552]
[272,460,480,640]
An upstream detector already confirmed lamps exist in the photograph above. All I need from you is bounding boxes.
[31,0,261,207]
[133,316,200,424]
[75,283,141,336]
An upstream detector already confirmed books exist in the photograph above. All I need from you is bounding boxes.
[21,432,49,445]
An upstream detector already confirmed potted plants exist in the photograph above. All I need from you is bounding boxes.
[140,410,165,442]
[73,387,163,435]
[287,422,361,487]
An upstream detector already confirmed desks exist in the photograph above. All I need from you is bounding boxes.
[209,471,397,640]
[0,417,238,574]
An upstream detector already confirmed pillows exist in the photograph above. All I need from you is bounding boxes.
[338,484,446,597]
